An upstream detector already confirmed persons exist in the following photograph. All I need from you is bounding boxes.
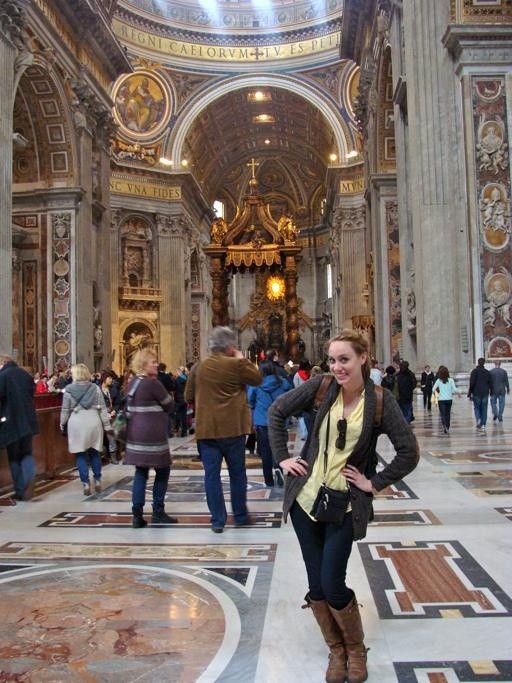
[432,366,462,434]
[433,365,444,394]
[126,348,177,528]
[311,362,335,378]
[293,358,312,440]
[467,358,493,432]
[381,365,400,405]
[490,360,509,421]
[420,365,434,410]
[46,373,60,393]
[0,354,39,501]
[32,349,329,377]
[98,372,122,464]
[370,359,384,386]
[174,365,187,436]
[396,363,414,424]
[246,359,293,490]
[35,375,47,395]
[401,361,416,421]
[267,328,419,683]
[60,363,115,496]
[157,362,174,438]
[184,326,263,533]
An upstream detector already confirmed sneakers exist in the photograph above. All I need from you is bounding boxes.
[275,468,284,488]
[264,488,274,499]
[476,419,481,427]
[482,423,487,428]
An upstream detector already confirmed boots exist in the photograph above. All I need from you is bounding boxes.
[328,588,369,683]
[132,506,147,528]
[303,593,348,683]
[150,503,177,523]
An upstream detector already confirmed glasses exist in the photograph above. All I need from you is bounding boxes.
[335,419,347,450]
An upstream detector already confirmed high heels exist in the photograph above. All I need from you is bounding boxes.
[95,480,101,493]
[83,484,90,496]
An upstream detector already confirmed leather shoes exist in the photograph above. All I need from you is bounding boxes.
[493,414,498,420]
[498,417,502,422]
[10,492,23,500]
[447,425,450,430]
[22,480,34,500]
[234,515,256,527]
[211,522,223,533]
[444,428,448,433]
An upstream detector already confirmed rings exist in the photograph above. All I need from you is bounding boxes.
[296,455,301,463]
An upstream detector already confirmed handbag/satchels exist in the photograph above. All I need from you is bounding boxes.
[421,374,428,391]
[310,404,353,526]
[63,385,92,436]
[112,377,144,444]
[392,374,400,402]
